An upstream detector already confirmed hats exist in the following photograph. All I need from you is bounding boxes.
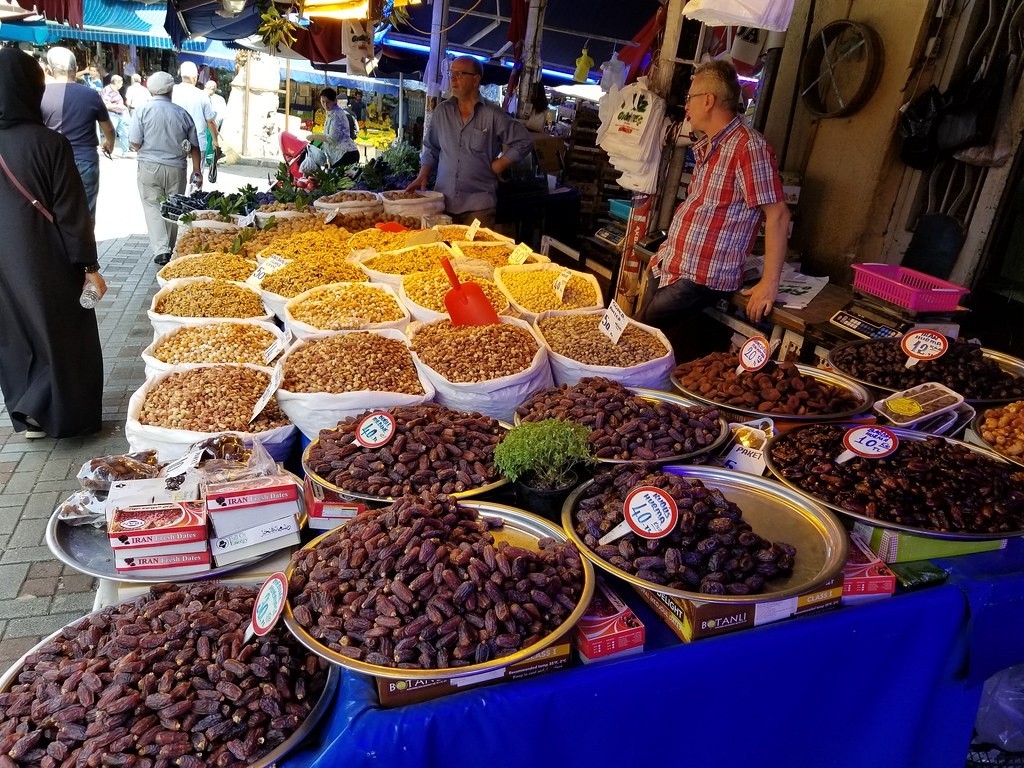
[180,61,198,78]
[147,70,174,95]
[47,46,76,71]
[336,93,347,99]
[205,80,217,89]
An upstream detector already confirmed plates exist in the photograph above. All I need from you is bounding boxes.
[45,462,310,583]
[514,385,734,466]
[301,411,527,502]
[671,355,874,420]
[971,403,1024,469]
[762,423,1024,542]
[279,498,595,680]
[0,580,341,768]
[561,467,848,603]
[826,337,1024,406]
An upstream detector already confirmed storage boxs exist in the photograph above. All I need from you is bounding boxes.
[629,573,845,644]
[850,262,972,311]
[573,577,646,659]
[841,530,896,596]
[571,643,644,665]
[842,593,892,606]
[839,514,1009,564]
[103,465,371,578]
[375,636,573,709]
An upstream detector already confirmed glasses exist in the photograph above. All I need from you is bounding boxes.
[447,70,478,80]
[682,91,711,103]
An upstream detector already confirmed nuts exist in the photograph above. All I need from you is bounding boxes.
[174,190,429,253]
[1,334,1024,768]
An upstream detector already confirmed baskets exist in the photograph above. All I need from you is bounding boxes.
[850,261,971,313]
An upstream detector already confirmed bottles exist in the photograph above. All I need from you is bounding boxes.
[190,173,202,194]
[80,273,104,308]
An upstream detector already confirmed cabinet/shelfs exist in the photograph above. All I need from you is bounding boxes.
[555,97,633,230]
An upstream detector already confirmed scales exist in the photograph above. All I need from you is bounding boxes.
[827,279,972,342]
[592,211,630,248]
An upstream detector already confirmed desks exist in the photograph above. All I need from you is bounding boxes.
[633,244,859,359]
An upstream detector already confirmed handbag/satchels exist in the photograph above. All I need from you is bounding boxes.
[208,146,226,183]
[894,78,1013,171]
[299,144,327,175]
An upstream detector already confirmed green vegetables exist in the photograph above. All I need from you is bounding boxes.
[210,141,420,222]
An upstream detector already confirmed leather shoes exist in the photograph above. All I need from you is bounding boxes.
[155,253,171,263]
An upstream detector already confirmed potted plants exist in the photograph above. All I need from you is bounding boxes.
[492,416,603,523]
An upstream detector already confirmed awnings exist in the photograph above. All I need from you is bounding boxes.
[1,0,207,51]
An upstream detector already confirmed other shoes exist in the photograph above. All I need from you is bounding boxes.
[122,154,133,158]
[25,421,48,438]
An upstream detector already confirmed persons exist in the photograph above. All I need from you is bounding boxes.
[171,61,220,196]
[632,60,791,366]
[127,71,203,264]
[403,55,532,231]
[204,80,226,165]
[75,64,104,96]
[99,74,132,158]
[40,46,115,230]
[0,47,107,442]
[99,66,127,100]
[126,73,152,114]
[348,91,373,122]
[389,89,409,139]
[306,87,359,169]
[38,61,57,85]
